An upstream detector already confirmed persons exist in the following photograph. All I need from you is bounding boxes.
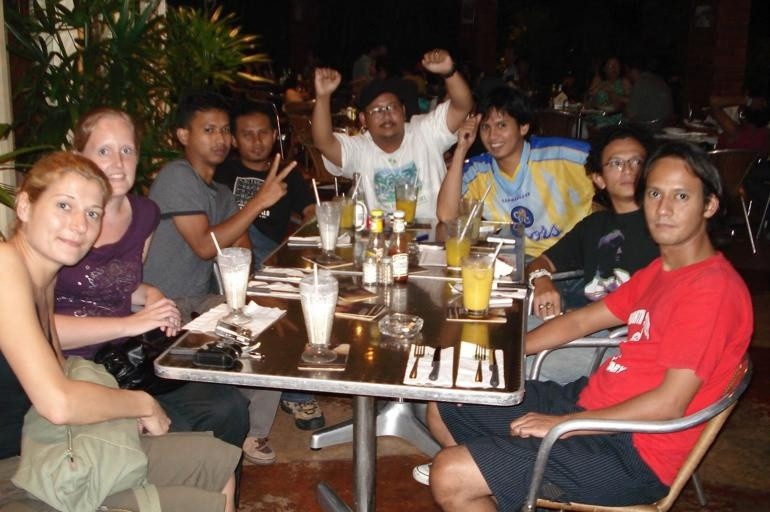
[137,96,325,464]
[408,127,756,511]
[280,38,474,221]
[0,110,237,512]
[709,78,768,153]
[437,43,674,267]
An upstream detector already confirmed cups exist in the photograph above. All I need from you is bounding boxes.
[392,184,418,227]
[315,201,343,263]
[333,196,354,243]
[444,217,471,267]
[218,248,253,324]
[300,276,337,363]
[457,197,483,245]
[460,253,497,318]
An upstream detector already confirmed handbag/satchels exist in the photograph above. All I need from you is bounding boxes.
[94,328,190,398]
[8,354,151,512]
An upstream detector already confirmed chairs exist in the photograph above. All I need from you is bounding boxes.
[522,338,752,512]
[527,269,629,379]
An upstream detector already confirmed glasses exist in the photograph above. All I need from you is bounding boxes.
[364,103,403,115]
[600,157,645,170]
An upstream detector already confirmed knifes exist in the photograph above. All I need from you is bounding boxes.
[489,345,499,386]
[429,346,440,380]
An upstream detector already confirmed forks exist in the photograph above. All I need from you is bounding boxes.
[475,344,485,381]
[409,342,425,379]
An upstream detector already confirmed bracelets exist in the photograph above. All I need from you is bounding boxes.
[745,95,753,108]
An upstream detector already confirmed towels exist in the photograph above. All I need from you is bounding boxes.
[455,341,506,390]
[181,300,287,340]
[452,280,526,299]
[403,343,454,388]
[254,265,305,283]
[246,280,301,301]
[486,252,517,280]
[287,235,350,247]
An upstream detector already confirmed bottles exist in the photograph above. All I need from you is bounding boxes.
[363,209,409,286]
[548,83,557,110]
[365,286,408,349]
[458,322,490,354]
[556,83,563,108]
[350,172,369,234]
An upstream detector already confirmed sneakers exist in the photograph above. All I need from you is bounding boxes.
[242,436,277,468]
[280,398,326,431]
[412,462,431,488]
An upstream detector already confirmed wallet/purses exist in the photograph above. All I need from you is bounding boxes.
[193,339,245,372]
